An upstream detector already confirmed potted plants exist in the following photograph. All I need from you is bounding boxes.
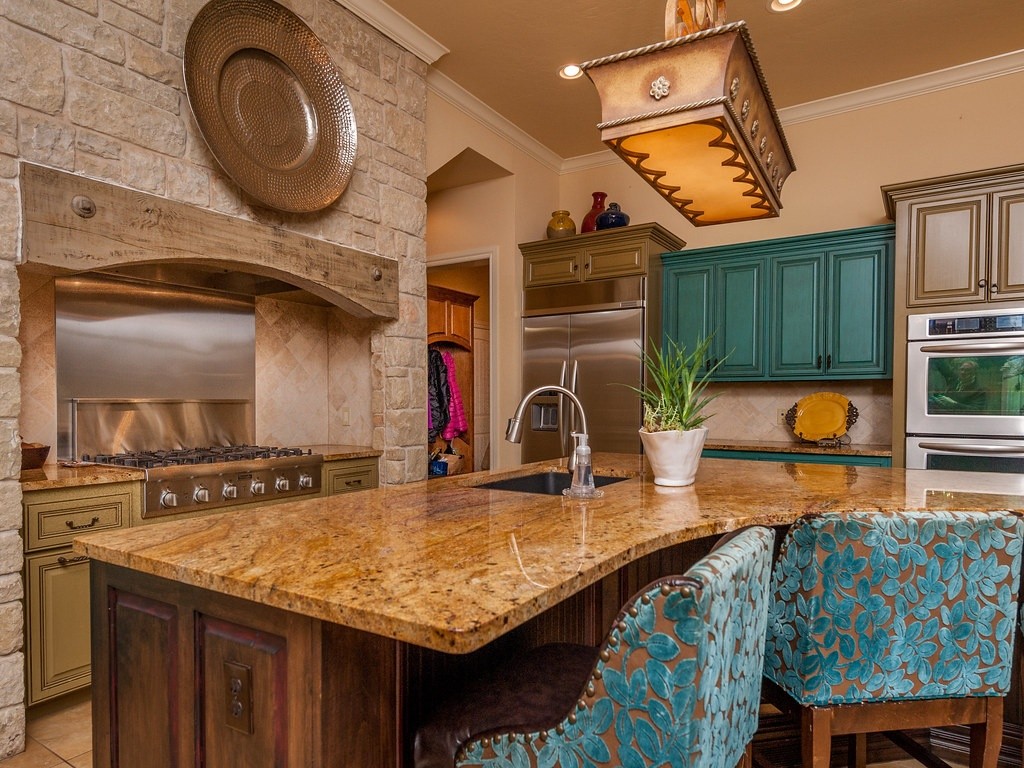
[606,326,736,486]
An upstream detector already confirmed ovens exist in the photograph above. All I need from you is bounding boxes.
[905,468,1024,508]
[906,308,1024,473]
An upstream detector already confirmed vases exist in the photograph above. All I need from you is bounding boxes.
[547,210,576,238]
[595,203,630,229]
[581,191,607,233]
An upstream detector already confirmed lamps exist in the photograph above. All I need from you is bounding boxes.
[580,0,796,227]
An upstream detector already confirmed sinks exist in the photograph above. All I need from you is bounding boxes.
[472,472,632,496]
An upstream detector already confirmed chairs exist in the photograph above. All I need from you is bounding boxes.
[411,523,776,768]
[753,509,1024,768]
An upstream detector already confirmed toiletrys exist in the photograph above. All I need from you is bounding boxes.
[569,433,598,500]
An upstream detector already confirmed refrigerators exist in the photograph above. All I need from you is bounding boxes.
[520,277,645,466]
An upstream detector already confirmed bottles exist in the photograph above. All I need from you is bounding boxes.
[596,202,630,230]
[581,191,608,233]
[547,210,576,238]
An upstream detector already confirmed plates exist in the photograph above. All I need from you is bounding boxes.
[785,391,858,441]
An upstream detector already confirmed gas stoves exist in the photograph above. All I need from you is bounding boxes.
[71,398,324,519]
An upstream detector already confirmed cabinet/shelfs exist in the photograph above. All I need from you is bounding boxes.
[24,491,133,707]
[526,242,645,287]
[906,187,1024,307]
[326,463,378,495]
[663,240,894,383]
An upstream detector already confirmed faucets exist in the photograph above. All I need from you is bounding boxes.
[504,385,592,492]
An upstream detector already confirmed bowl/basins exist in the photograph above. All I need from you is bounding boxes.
[22,446,50,469]
